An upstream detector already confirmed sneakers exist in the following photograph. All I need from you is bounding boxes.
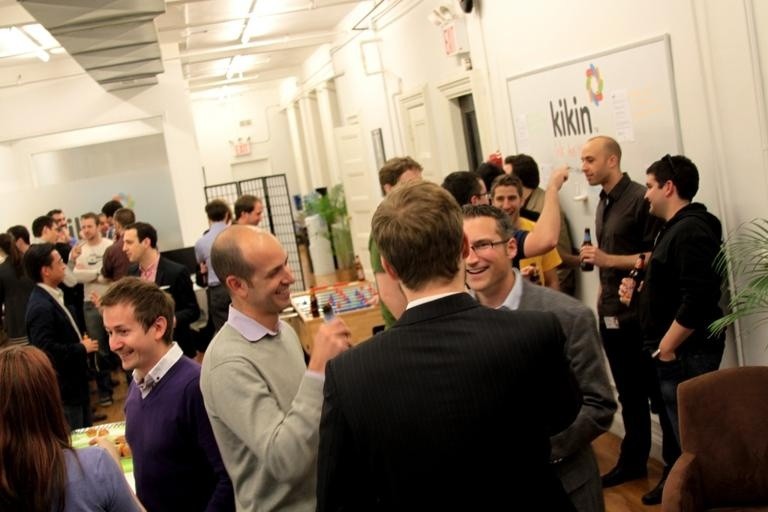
[101,395,111,407]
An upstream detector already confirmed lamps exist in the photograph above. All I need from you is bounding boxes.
[9,25,50,63]
[225,34,249,80]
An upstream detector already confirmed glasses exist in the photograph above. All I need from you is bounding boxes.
[53,256,64,268]
[470,237,511,253]
[474,190,493,200]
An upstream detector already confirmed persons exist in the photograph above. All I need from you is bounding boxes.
[33,216,58,244]
[201,223,351,512]
[317,182,581,512]
[502,155,572,297]
[101,278,236,512]
[195,201,233,334]
[231,196,263,226]
[7,226,30,254]
[441,167,569,272]
[24,244,99,430]
[617,156,731,505]
[491,176,563,292]
[0,234,35,345]
[68,213,134,407]
[557,136,681,505]
[102,208,138,280]
[0,344,147,512]
[370,158,424,329]
[48,210,77,246]
[103,201,123,241]
[462,204,617,512]
[123,221,199,359]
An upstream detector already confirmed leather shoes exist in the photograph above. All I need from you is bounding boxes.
[109,379,118,385]
[600,462,647,487]
[641,473,666,504]
[93,413,109,423]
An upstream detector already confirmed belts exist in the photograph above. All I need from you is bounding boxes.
[597,314,640,328]
[208,285,223,290]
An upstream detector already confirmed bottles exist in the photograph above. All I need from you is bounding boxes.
[311,285,320,317]
[322,302,353,349]
[579,228,594,272]
[354,255,365,281]
[618,253,645,307]
[529,262,542,287]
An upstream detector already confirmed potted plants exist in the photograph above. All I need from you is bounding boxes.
[300,184,356,283]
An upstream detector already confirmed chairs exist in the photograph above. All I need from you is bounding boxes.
[659,364,768,511]
